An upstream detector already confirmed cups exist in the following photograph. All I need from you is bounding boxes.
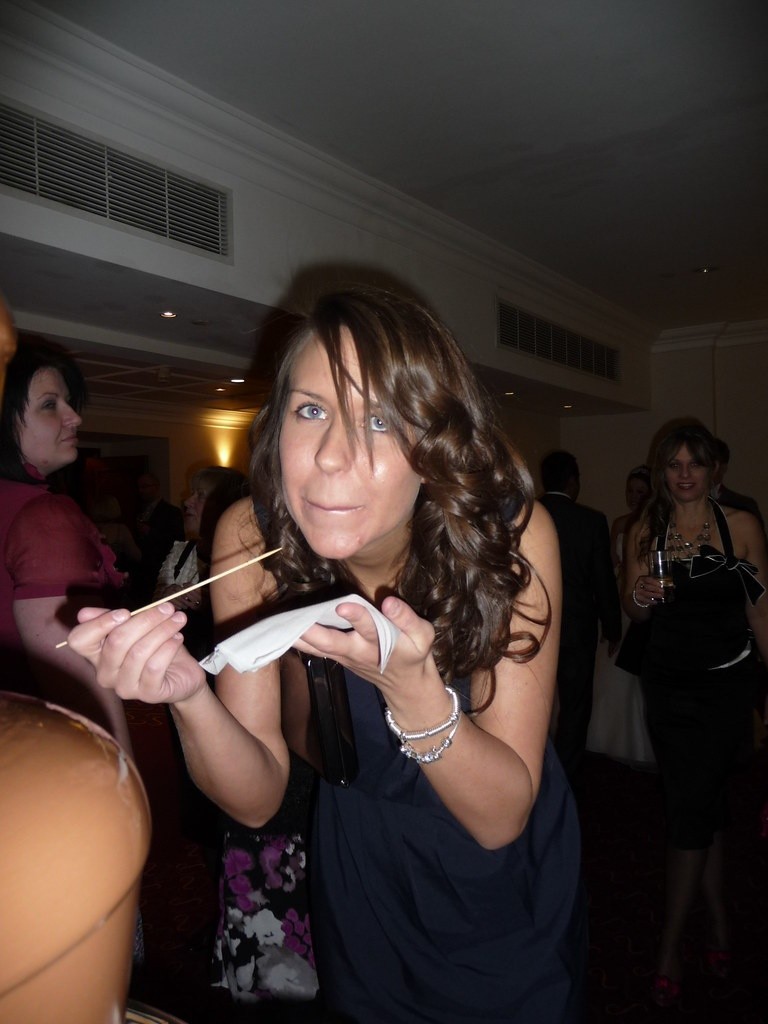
[647,550,675,603]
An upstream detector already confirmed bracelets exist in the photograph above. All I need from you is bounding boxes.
[632,589,649,608]
[384,686,460,762]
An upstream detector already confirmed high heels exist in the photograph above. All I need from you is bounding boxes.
[709,925,736,979]
[648,934,681,1007]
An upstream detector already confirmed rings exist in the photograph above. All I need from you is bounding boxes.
[640,584,645,589]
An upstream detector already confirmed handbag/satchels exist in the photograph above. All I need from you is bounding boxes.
[210,784,325,1005]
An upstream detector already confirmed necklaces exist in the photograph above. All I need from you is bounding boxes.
[667,518,711,568]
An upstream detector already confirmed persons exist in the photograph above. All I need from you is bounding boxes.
[0,330,319,1024]
[624,416,768,1007]
[70,280,583,1024]
[585,464,657,774]
[535,449,623,796]
